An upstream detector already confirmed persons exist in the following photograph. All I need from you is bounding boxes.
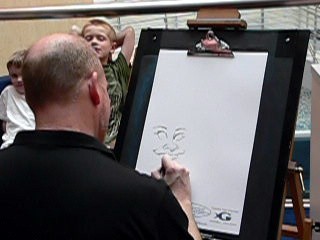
[71,20,135,151]
[0,34,202,240]
[0,50,36,149]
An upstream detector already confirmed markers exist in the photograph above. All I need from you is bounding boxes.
[158,161,169,181]
